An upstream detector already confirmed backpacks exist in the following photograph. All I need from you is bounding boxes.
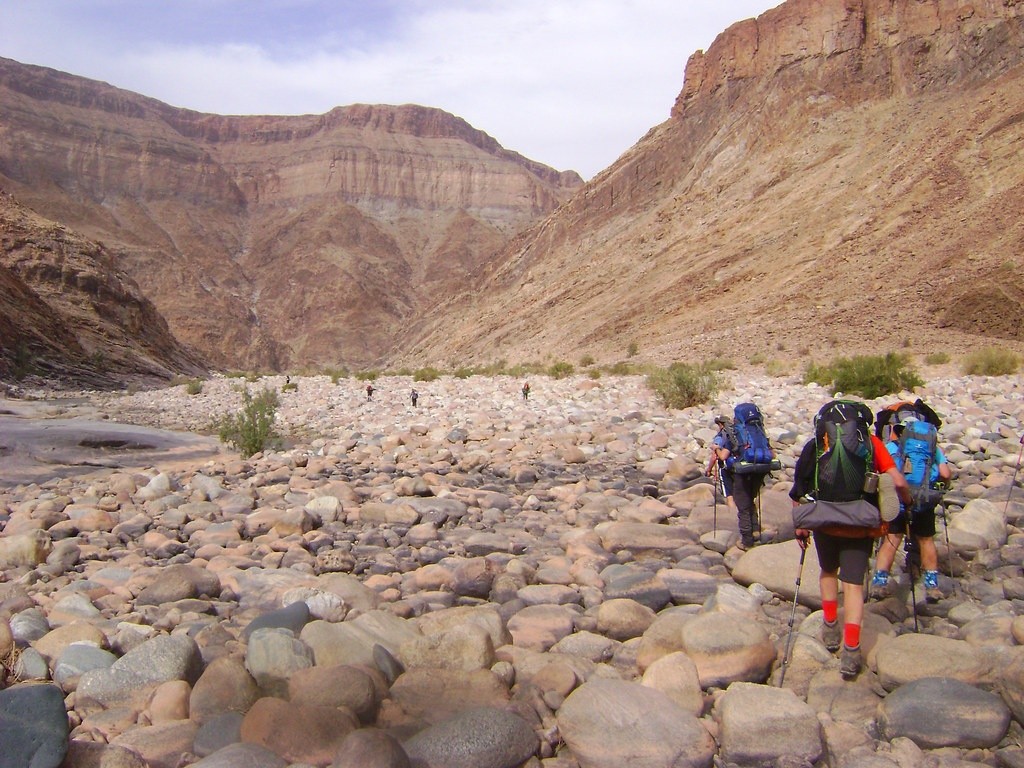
[813,399,876,502]
[732,402,772,463]
[893,421,938,487]
[882,409,924,445]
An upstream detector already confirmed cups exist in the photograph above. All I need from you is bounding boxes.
[863,471,879,494]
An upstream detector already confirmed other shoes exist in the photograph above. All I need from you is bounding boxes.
[925,588,945,600]
[869,584,885,598]
[735,541,750,551]
[878,472,902,522]
[821,619,843,649]
[752,531,760,540]
[839,642,861,675]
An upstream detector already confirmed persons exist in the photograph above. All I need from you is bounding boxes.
[792,395,915,676]
[706,408,766,551]
[872,401,953,589]
[368,386,376,402]
[523,381,531,399]
[410,389,416,408]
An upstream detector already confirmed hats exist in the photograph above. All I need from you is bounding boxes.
[893,416,919,439]
[713,415,730,423]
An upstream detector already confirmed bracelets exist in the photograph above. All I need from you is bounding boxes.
[902,496,914,506]
